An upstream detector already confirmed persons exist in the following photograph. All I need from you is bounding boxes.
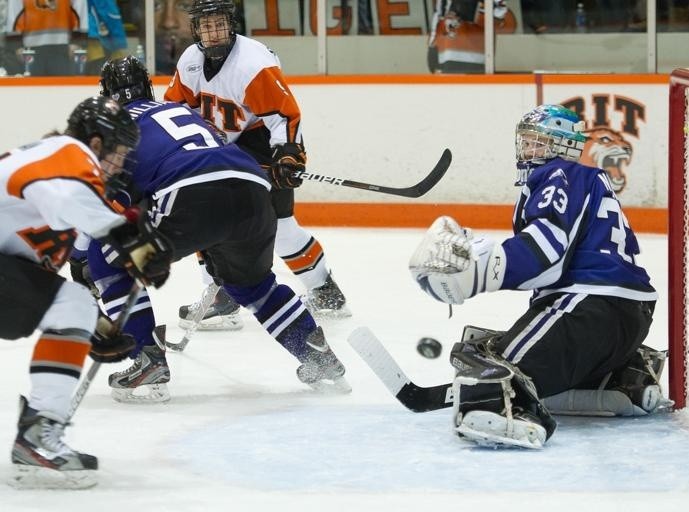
[427,0,516,74]
[164,1,354,329]
[0,0,130,76]
[68,53,355,405]
[0,96,169,490]
[408,105,676,452]
[136,0,197,75]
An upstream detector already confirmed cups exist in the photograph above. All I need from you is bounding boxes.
[22,50,36,77]
[72,50,88,74]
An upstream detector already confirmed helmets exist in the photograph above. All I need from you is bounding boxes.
[514,104,588,190]
[185,0,238,63]
[62,95,140,191]
[98,54,154,108]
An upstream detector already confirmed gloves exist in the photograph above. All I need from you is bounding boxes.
[266,142,306,192]
[408,215,507,320]
[121,232,171,287]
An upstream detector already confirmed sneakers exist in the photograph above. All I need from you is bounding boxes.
[10,394,98,472]
[108,341,170,390]
[178,285,241,322]
[456,391,558,443]
[617,379,660,413]
[296,327,346,384]
[297,268,346,313]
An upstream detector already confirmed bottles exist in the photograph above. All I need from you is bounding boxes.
[136,45,145,66]
[576,3,587,33]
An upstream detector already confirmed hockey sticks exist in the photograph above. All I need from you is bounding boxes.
[346,322,453,414]
[164,281,223,356]
[254,148,452,198]
